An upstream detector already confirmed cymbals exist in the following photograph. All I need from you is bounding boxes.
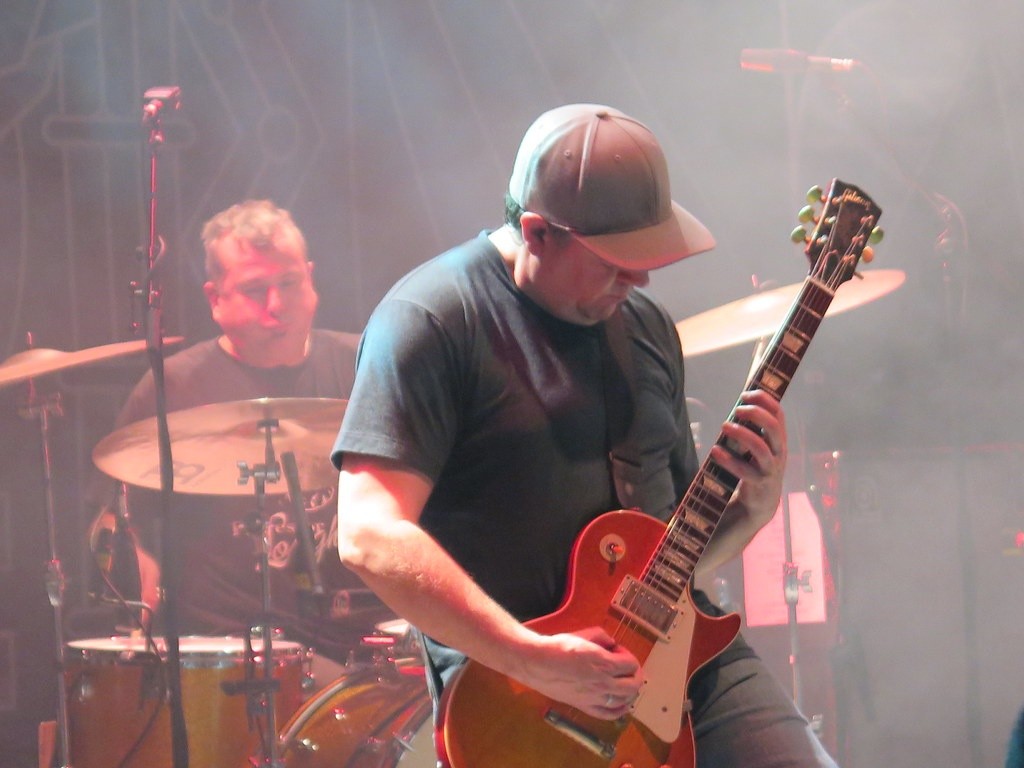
[674,268,905,357]
[91,396,350,498]
[0,334,188,384]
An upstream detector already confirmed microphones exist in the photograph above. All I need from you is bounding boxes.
[142,87,182,122]
[89,595,148,611]
[739,49,862,73]
[280,452,325,597]
[115,484,133,543]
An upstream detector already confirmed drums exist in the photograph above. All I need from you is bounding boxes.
[59,635,304,768]
[365,615,428,666]
[252,658,441,768]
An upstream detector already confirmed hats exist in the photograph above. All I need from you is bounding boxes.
[510,103,716,271]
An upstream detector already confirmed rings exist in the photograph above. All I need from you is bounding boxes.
[604,695,615,709]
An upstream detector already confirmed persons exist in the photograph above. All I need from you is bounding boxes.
[113,199,404,666]
[329,103,841,768]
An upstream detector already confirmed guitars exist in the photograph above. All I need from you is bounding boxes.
[433,177,886,768]
